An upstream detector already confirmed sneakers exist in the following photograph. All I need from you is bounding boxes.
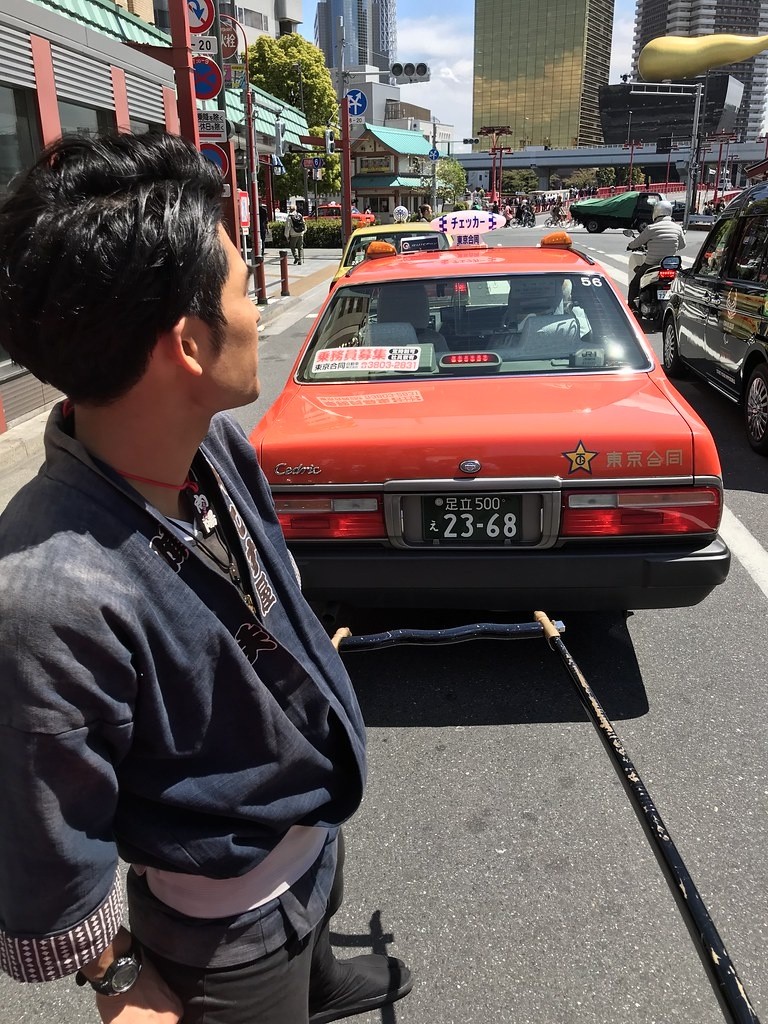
[308,954,415,1021]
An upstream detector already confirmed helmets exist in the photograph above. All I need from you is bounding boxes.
[652,201,673,222]
[721,198,725,201]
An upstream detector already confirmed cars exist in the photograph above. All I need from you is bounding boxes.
[669,201,694,221]
[303,205,375,228]
[248,241,731,611]
[329,222,455,293]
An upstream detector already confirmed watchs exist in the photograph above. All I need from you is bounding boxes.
[75,932,143,997]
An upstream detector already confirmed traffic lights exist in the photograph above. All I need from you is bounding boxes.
[390,62,428,79]
[325,130,335,154]
[463,138,479,144]
[275,122,287,157]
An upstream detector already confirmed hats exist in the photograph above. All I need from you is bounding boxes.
[290,205,295,210]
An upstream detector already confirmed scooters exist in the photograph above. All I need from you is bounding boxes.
[623,230,683,326]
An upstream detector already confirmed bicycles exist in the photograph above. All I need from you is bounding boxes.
[544,211,572,229]
[509,211,536,229]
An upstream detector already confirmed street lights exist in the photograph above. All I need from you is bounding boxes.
[525,117,529,147]
[628,111,633,142]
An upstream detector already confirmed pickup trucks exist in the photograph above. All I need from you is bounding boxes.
[570,192,676,233]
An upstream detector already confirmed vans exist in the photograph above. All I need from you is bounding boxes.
[718,178,732,191]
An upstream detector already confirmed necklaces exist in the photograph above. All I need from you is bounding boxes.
[112,468,219,537]
[161,497,257,615]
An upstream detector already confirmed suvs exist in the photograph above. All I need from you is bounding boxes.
[663,174,768,455]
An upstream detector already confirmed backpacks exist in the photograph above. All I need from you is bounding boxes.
[288,212,305,233]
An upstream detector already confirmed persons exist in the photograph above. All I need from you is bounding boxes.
[471,185,636,225]
[249,198,269,258]
[703,198,725,214]
[627,200,687,314]
[418,204,432,222]
[352,203,386,215]
[0,135,367,1024]
[285,206,307,265]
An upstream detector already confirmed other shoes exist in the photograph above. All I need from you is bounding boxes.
[294,258,299,264]
[298,262,301,265]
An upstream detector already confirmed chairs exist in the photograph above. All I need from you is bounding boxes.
[371,284,450,354]
